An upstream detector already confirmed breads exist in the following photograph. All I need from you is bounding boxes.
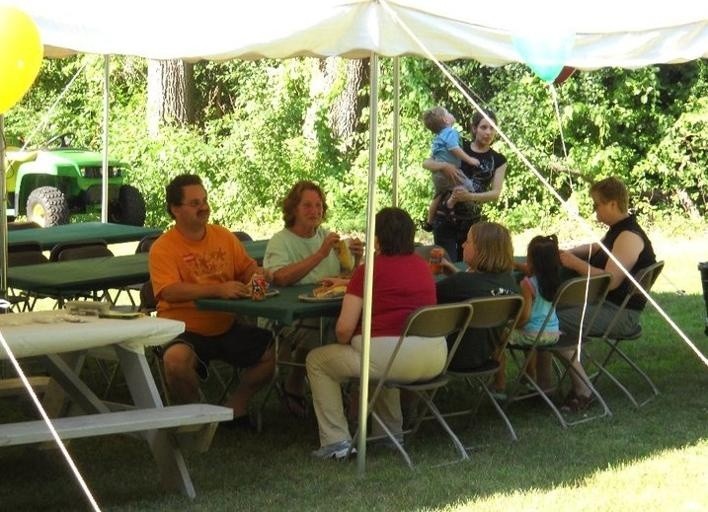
[333,238,355,273]
[312,285,346,298]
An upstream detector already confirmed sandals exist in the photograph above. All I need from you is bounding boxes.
[436,200,457,224]
[420,219,433,232]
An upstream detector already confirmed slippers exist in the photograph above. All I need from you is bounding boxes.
[481,384,507,400]
[282,380,311,419]
[561,391,596,412]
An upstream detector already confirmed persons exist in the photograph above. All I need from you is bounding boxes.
[430,109,507,264]
[302,207,447,461]
[147,173,277,435]
[558,178,658,412]
[489,232,565,402]
[344,221,519,438]
[423,106,483,231]
[263,180,363,420]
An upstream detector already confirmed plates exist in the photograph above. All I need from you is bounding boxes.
[243,288,280,297]
[298,292,345,302]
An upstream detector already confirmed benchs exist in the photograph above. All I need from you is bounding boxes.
[2,402,235,498]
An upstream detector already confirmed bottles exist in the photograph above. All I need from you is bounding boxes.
[430,247,445,281]
[446,186,462,209]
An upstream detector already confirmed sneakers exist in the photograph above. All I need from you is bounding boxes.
[311,442,356,460]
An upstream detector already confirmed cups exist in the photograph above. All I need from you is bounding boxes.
[333,238,355,269]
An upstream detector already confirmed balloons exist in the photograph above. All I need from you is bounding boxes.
[527,62,567,82]
[1,5,45,121]
[554,66,577,86]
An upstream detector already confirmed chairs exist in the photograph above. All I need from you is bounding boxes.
[336,301,474,470]
[485,270,615,430]
[2,220,271,392]
[553,260,664,406]
[410,294,524,463]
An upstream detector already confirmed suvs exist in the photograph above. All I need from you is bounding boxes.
[3,132,147,228]
